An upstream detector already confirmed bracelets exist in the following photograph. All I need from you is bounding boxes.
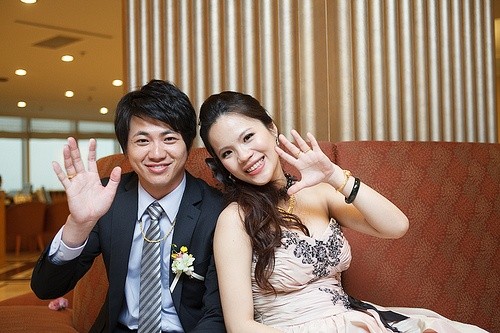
[344,177,360,204]
[335,169,350,193]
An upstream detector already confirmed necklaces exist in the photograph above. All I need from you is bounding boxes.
[275,189,297,229]
[139,220,176,243]
[274,172,292,202]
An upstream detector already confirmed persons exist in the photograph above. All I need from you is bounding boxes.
[25,80,237,333]
[199,91,491,333]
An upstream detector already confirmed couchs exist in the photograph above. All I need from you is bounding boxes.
[0,140,500,333]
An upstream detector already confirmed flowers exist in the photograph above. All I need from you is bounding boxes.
[168,243,204,293]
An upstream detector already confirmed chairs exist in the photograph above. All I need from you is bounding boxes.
[6,199,72,258]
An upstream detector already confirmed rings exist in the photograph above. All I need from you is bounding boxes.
[67,173,77,179]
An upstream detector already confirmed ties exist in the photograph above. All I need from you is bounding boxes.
[137,203,164,333]
